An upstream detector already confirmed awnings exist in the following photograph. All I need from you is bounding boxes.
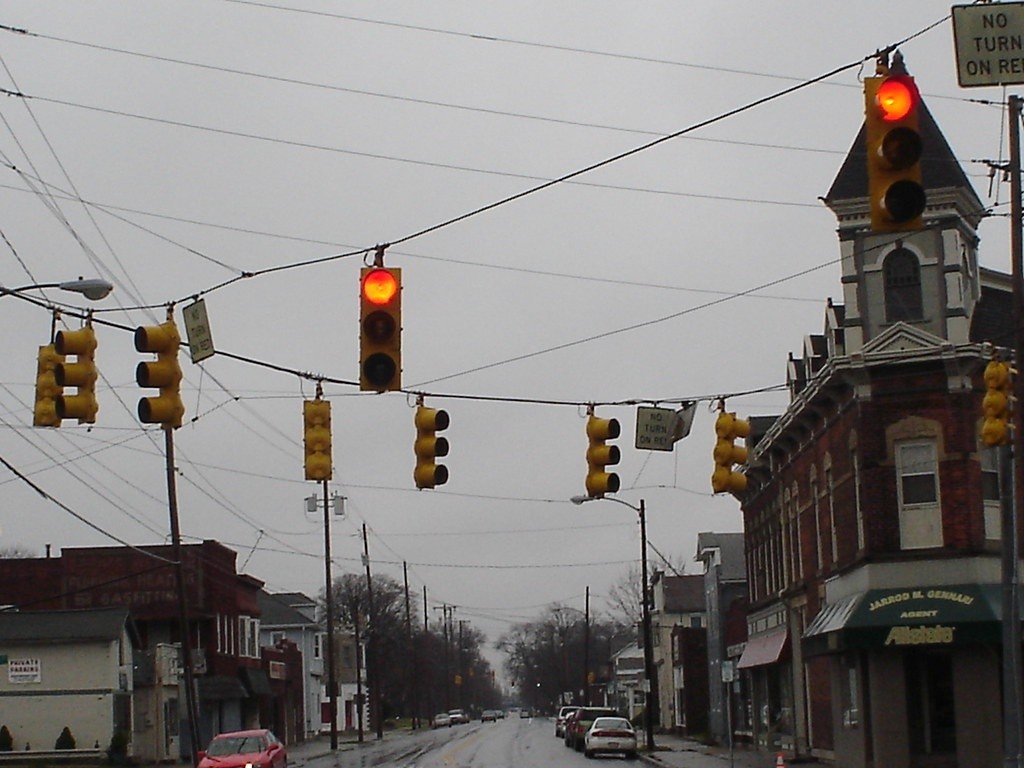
[736,629,792,670]
[798,585,1024,639]
[241,668,272,698]
[205,676,249,701]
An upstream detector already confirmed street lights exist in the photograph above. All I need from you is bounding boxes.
[552,607,589,706]
[571,495,669,752]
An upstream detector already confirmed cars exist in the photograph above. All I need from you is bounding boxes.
[739,704,768,728]
[448,709,471,725]
[432,714,451,729]
[197,728,288,768]
[584,716,640,760]
[843,708,858,727]
[494,707,535,720]
[552,706,587,738]
[481,710,497,722]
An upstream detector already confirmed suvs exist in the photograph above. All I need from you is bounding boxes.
[562,707,621,753]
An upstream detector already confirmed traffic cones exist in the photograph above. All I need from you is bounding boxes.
[776,751,785,768]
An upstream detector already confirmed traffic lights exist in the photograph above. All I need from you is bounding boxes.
[984,362,1022,446]
[711,411,751,493]
[358,267,403,392]
[585,416,621,496]
[413,406,449,491]
[865,76,927,233]
[54,327,98,424]
[134,323,183,430]
